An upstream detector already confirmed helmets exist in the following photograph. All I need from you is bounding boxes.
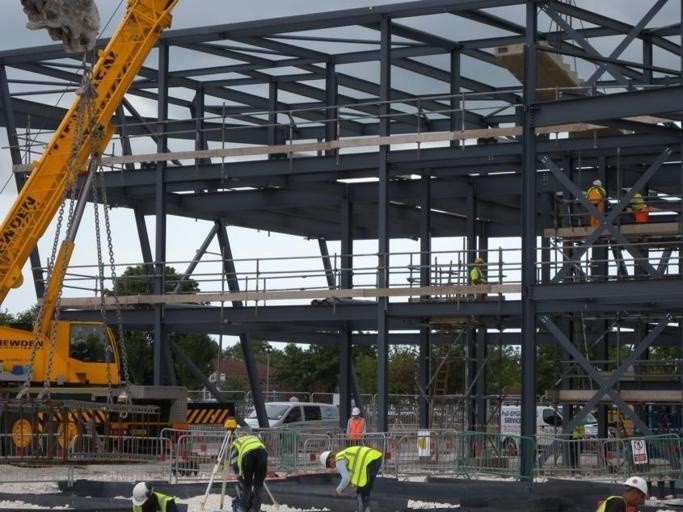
[319,450,333,469]
[351,407,361,416]
[474,258,485,265]
[593,180,602,187]
[130,481,154,507]
[622,475,648,497]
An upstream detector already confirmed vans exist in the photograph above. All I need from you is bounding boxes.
[500,400,610,456]
[237,402,339,447]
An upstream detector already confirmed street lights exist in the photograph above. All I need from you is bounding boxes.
[265,344,272,402]
[196,248,226,389]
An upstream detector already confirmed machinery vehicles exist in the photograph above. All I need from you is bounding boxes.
[0,1,178,453]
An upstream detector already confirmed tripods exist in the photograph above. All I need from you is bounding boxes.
[199,428,280,512]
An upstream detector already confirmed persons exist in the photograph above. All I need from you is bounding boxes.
[129,480,180,512]
[344,406,366,445]
[586,179,607,227]
[469,257,488,301]
[319,445,382,511]
[629,193,648,224]
[228,433,269,511]
[594,474,648,512]
